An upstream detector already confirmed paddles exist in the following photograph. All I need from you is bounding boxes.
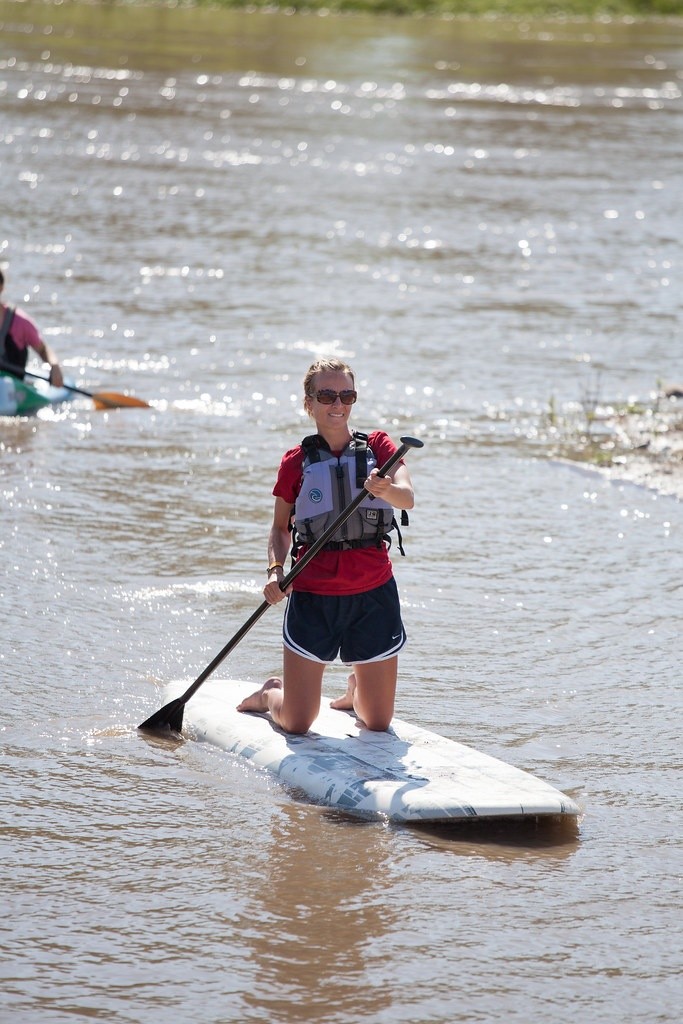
[0,360,150,411]
[138,435,421,736]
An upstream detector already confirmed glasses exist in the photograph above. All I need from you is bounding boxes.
[309,389,357,405]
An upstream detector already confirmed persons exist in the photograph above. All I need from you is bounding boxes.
[0,271,64,387]
[234,357,417,736]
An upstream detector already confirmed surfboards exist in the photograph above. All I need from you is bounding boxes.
[162,673,583,825]
